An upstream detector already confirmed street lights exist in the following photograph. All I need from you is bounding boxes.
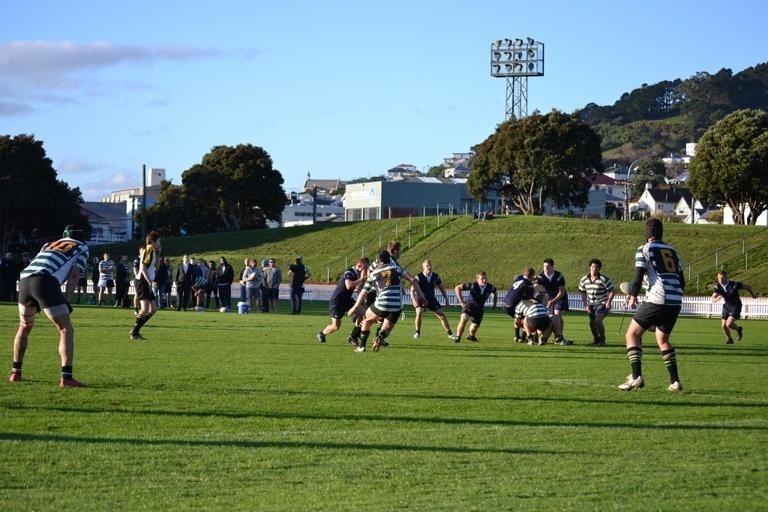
[625,152,657,221]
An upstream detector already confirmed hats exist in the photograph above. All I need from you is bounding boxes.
[262,259,268,266]
[270,258,274,263]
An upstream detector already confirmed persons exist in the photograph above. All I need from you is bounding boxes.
[471,207,496,222]
[4,220,95,387]
[318,239,618,353]
[127,229,162,341]
[3,251,32,302]
[78,248,145,307]
[613,217,688,392]
[151,254,234,312]
[709,268,759,345]
[238,257,282,313]
[286,257,311,315]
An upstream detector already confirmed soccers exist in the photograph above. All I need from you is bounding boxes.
[220,306,228,313]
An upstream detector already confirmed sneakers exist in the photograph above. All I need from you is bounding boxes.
[130,330,143,340]
[60,376,87,387]
[617,374,644,391]
[10,373,21,382]
[666,381,682,392]
